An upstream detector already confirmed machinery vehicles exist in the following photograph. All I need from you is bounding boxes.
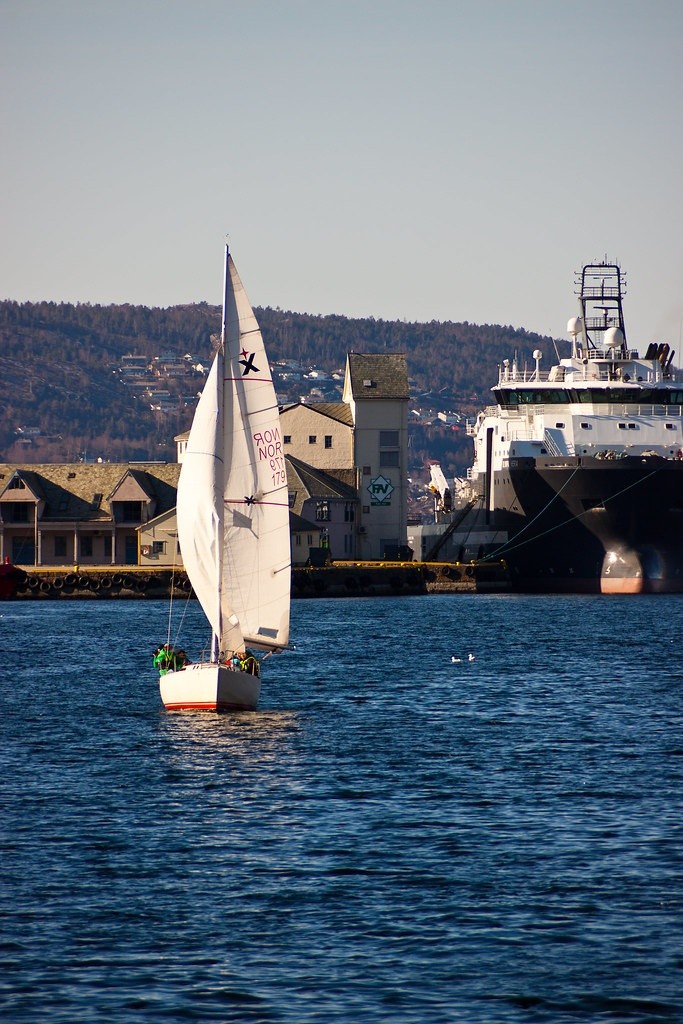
[423,495,485,562]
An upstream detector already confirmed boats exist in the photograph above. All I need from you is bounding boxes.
[406,263,683,593]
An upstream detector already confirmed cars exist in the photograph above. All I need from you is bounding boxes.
[16,427,43,440]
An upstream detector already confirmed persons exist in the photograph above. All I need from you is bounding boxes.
[153,643,192,676]
[218,649,259,677]
[594,448,628,460]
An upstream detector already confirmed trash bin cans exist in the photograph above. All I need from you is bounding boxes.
[308,547,332,568]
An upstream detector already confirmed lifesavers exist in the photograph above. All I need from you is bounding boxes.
[464,565,474,577]
[18,572,148,594]
[168,575,193,592]
[441,566,451,576]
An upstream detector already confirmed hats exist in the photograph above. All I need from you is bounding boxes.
[177,649,187,655]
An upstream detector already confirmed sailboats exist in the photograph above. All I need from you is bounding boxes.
[159,244,297,713]
